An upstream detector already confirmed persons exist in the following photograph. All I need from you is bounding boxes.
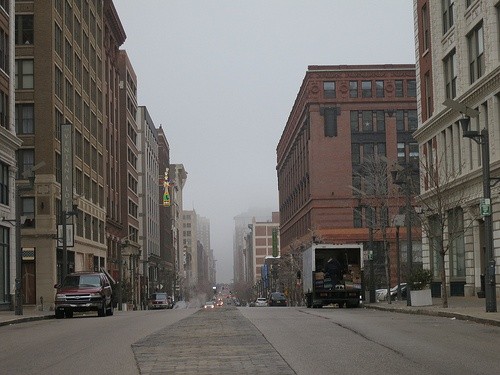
[326,257,340,289]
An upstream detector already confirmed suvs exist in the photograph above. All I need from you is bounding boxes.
[53,271,115,320]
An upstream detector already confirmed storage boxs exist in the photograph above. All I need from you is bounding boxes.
[315,272,362,289]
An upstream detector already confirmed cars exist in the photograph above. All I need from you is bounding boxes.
[202,291,249,309]
[383,281,423,301]
[253,297,268,307]
[146,292,172,310]
[267,292,288,307]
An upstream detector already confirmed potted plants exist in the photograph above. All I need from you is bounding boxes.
[407,270,433,306]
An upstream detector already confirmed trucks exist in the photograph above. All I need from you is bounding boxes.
[297,242,365,308]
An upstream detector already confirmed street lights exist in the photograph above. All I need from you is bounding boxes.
[118,238,130,311]
[60,195,80,278]
[463,126,497,312]
[392,178,414,307]
